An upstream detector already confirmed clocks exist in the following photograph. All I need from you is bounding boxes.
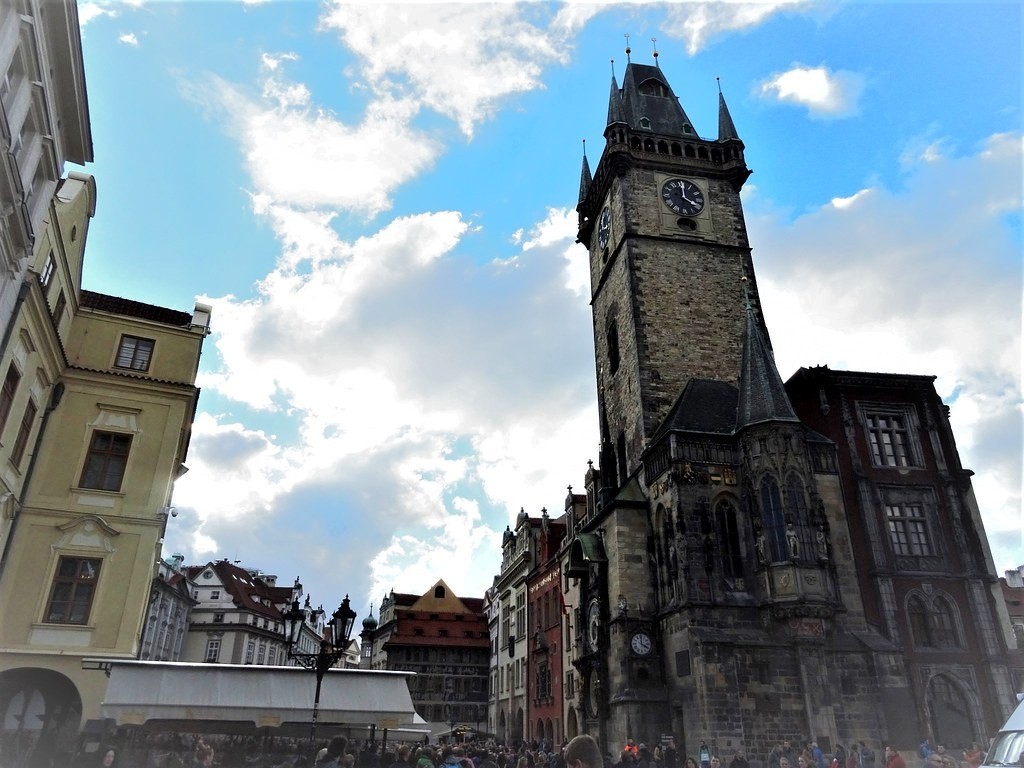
[631,633,651,655]
[598,207,611,250]
[662,179,705,215]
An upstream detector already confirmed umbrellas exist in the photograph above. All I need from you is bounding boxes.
[434,724,484,743]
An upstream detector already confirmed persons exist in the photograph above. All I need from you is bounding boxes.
[96,744,117,768]
[222,735,989,768]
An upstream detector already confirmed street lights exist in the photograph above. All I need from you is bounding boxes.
[279,593,358,767]
